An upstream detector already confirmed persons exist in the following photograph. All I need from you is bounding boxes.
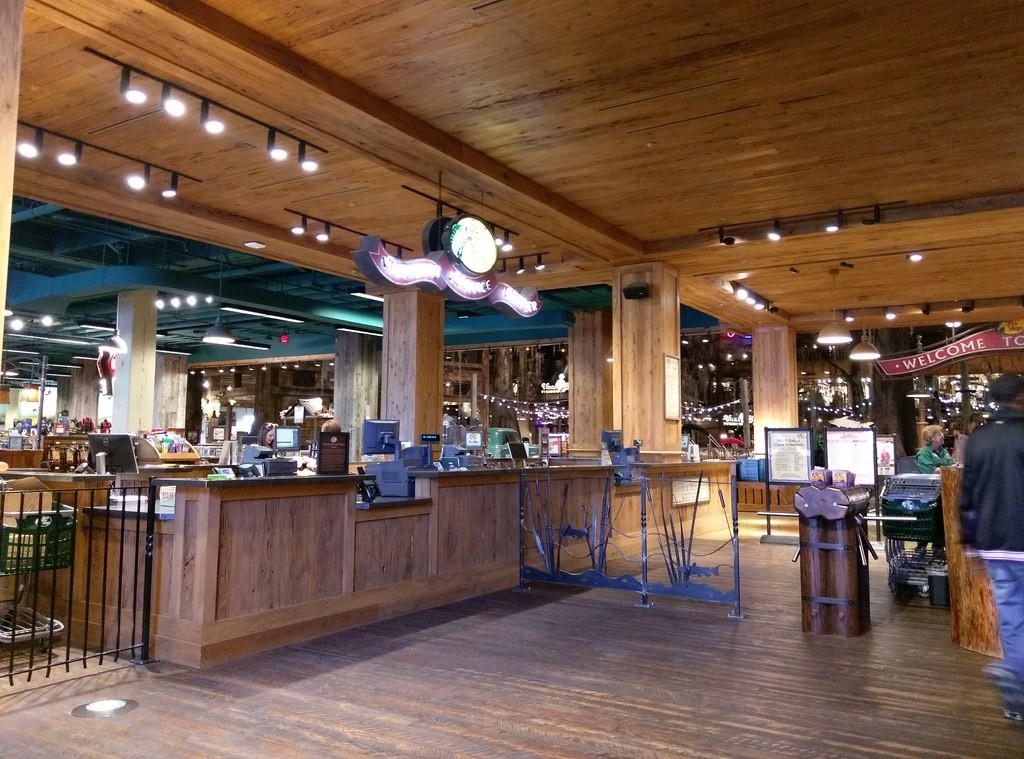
[257,422,278,449]
[59,410,76,430]
[951,425,969,464]
[915,424,959,474]
[301,420,341,471]
[956,374,1024,720]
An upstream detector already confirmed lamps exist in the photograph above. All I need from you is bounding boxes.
[718,206,980,420]
[4,45,568,373]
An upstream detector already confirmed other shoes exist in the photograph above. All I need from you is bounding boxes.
[1004,704,1024,722]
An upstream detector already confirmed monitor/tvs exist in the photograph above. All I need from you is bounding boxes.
[362,419,402,460]
[602,430,623,452]
[275,425,300,451]
[88,433,139,475]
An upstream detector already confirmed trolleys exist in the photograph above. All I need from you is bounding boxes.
[0,497,79,654]
[878,471,947,602]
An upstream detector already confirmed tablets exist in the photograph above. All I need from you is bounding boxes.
[507,443,528,460]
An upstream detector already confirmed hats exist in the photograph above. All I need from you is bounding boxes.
[990,373,1024,400]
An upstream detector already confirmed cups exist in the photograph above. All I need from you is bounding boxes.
[688,444,700,462]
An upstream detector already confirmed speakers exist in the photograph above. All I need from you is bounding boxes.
[623,283,649,300]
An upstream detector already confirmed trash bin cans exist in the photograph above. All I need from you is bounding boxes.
[927,566,950,607]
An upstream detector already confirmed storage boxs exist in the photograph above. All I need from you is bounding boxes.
[739,459,766,480]
[0,476,53,560]
